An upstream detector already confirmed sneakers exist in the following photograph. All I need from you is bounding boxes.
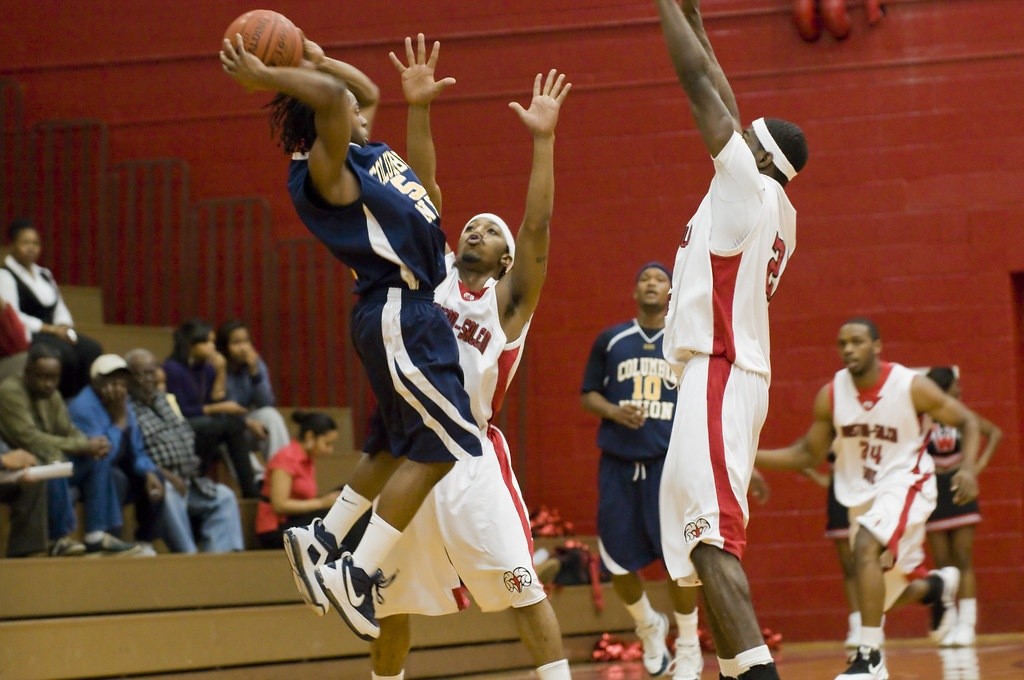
[48,538,87,557]
[666,636,704,680]
[87,530,141,556]
[922,566,961,642]
[929,620,976,645]
[282,517,350,617]
[834,646,888,680]
[314,550,381,641]
[634,613,671,676]
[844,611,862,648]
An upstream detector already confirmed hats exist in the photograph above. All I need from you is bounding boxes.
[89,353,128,379]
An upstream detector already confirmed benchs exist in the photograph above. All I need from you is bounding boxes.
[0,284,675,680]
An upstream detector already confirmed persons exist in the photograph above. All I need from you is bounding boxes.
[371,31,571,680]
[580,263,770,680]
[755,317,979,680]
[800,437,861,649]
[0,318,291,557]
[657,0,808,680]
[220,28,483,641]
[0,221,103,397]
[920,365,1002,646]
[256,409,345,549]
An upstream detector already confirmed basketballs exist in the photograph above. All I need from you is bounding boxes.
[222,9,304,68]
